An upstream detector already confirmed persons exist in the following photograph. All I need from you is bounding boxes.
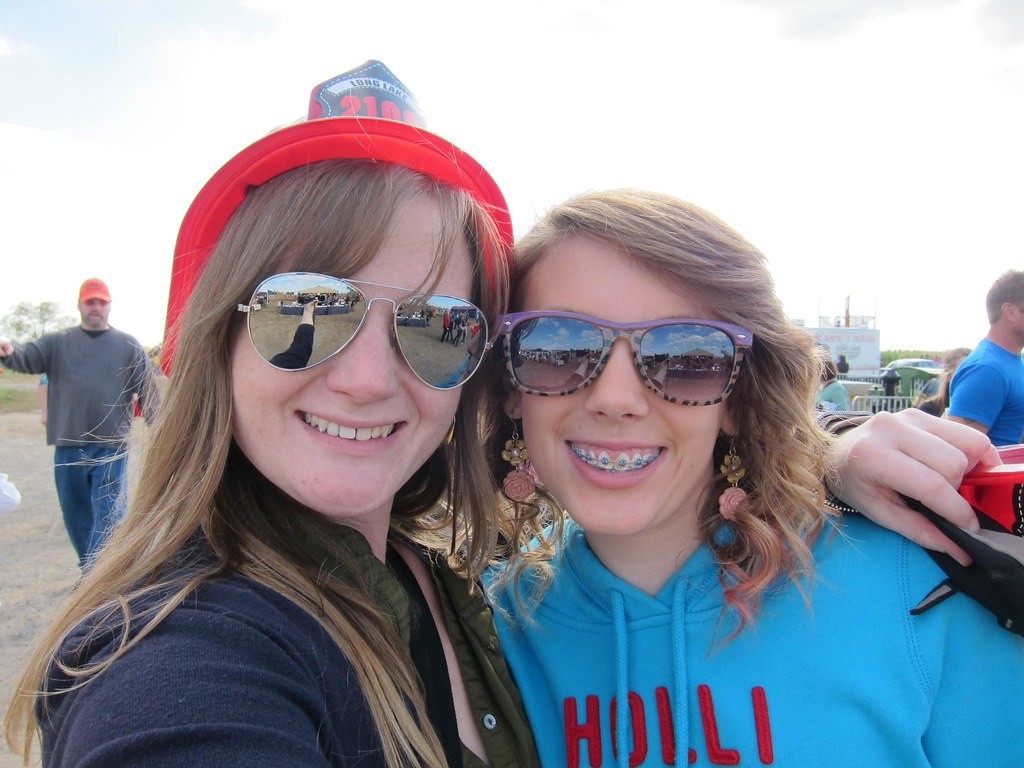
[419,303,485,377]
[565,347,596,392]
[263,293,359,370]
[648,351,674,390]
[38,117,1008,766]
[478,186,1024,767]
[810,268,1024,450]
[0,275,164,575]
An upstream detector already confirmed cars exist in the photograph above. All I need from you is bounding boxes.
[881,358,943,379]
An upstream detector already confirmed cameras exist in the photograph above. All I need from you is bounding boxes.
[576,349,589,357]
[297,294,315,304]
[656,354,668,363]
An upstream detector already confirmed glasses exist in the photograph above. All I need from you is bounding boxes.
[84,300,106,305]
[496,311,754,407]
[237,271,494,390]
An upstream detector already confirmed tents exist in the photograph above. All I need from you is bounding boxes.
[297,285,339,306]
[679,348,715,371]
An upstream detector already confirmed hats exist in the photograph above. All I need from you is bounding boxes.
[79,277,111,302]
[160,116,516,379]
